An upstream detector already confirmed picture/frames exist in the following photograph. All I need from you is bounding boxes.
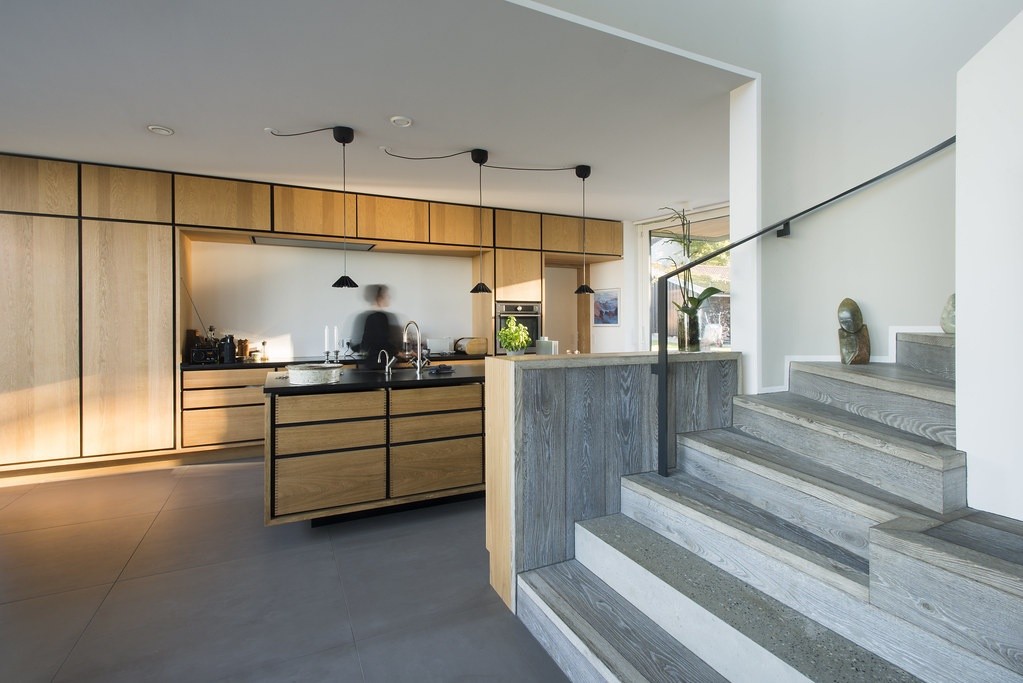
[592,287,620,326]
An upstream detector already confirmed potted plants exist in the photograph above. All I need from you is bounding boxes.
[497,316,532,356]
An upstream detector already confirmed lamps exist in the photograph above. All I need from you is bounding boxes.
[575,164,595,295]
[468,148,493,293]
[333,126,359,288]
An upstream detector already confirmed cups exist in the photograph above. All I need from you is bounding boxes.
[251,353,259,361]
[567,350,580,355]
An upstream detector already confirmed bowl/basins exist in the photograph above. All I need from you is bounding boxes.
[285,363,343,385]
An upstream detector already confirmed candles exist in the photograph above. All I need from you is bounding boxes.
[333,326,338,350]
[324,326,330,352]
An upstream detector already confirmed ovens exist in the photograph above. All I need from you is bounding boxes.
[495,302,542,355]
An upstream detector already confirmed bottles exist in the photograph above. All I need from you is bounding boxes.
[260,341,267,362]
[223,335,235,364]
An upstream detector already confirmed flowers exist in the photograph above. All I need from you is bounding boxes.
[656,206,724,319]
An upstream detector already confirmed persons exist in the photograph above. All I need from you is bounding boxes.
[350,284,418,369]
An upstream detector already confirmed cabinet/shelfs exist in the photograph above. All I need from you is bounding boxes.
[0,150,182,487]
[181,366,277,450]
[262,384,486,526]
[473,250,545,355]
[174,171,623,267]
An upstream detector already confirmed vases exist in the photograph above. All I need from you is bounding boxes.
[676,312,700,353]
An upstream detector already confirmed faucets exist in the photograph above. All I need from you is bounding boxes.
[385,373,392,382]
[403,322,431,374]
[377,350,397,373]
[417,374,423,380]
